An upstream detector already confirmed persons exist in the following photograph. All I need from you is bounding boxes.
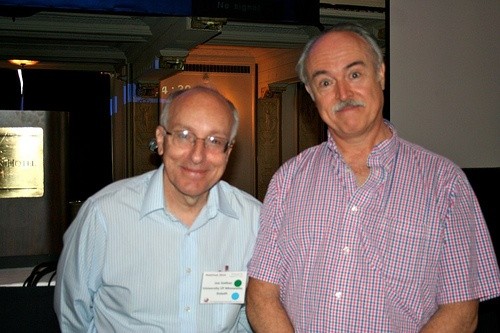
[54,86,263,333]
[245,22,500,333]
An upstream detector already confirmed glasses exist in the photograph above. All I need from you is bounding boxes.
[164,129,232,154]
